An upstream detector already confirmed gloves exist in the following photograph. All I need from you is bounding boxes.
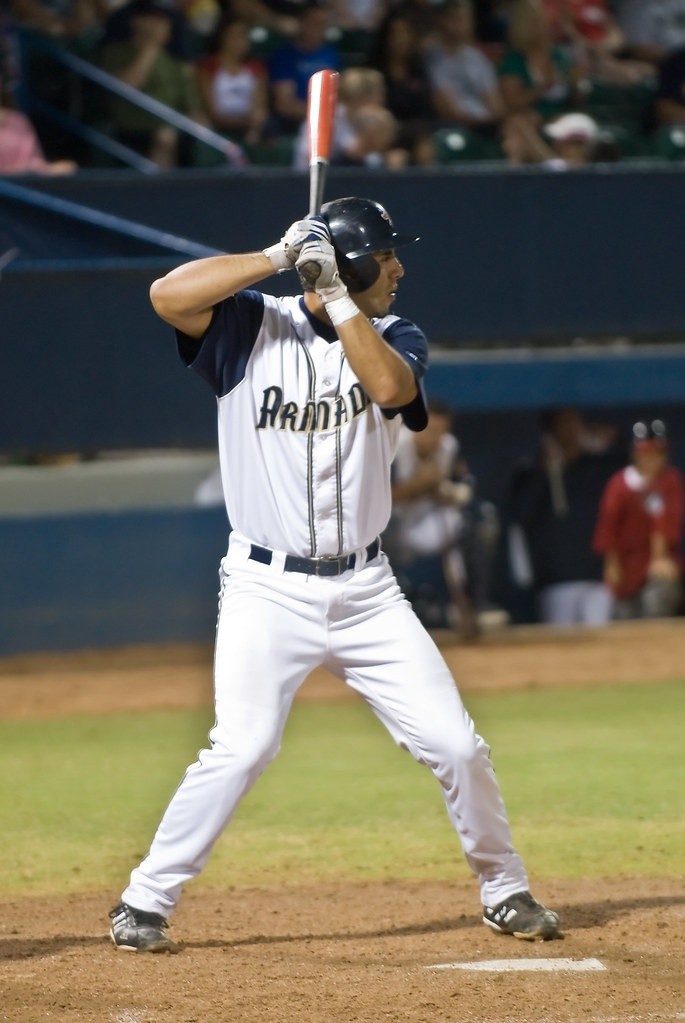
[295,240,348,304]
[265,216,332,270]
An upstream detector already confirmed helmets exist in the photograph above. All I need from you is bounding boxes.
[305,197,422,294]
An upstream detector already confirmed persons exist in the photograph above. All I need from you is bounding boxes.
[377,400,634,646]
[109,197,559,952]
[0,0,685,176]
[592,417,685,620]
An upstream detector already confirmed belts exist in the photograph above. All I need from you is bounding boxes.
[250,539,379,577]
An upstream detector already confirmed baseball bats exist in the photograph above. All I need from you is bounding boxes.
[300,69,341,282]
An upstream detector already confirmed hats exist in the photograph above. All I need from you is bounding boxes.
[546,115,597,143]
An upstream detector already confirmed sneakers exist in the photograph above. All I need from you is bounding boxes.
[108,905,171,953]
[484,891,560,941]
